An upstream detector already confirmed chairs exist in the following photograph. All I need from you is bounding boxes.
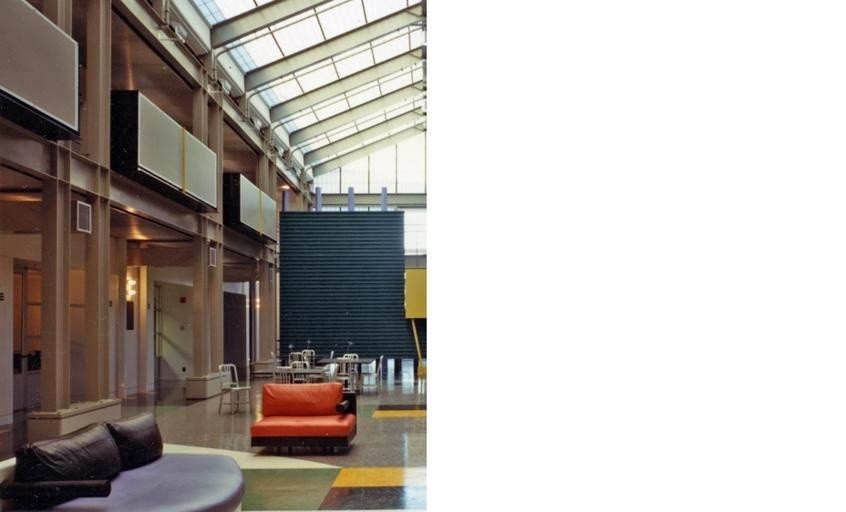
[217,362,248,418]
[270,347,384,400]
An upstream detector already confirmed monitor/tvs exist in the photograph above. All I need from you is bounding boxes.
[209,247,216,267]
[75,200,92,234]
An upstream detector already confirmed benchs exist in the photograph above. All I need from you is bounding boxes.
[249,383,357,456]
[0,410,248,512]
